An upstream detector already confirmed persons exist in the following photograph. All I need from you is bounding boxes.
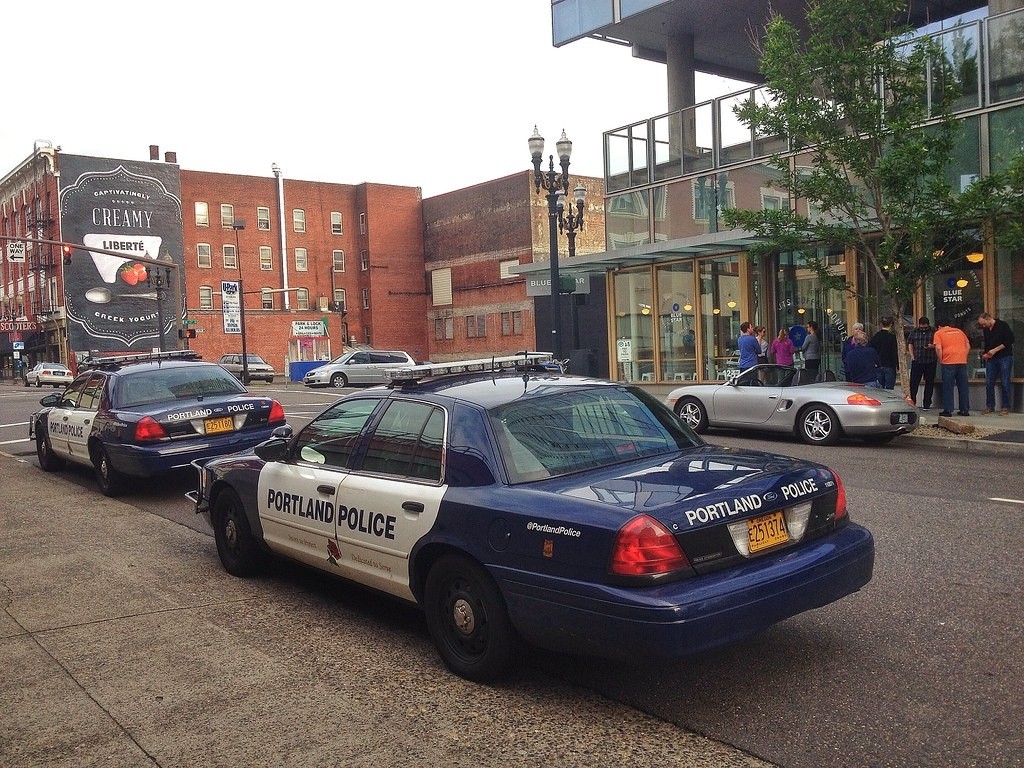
[979,313,1014,416]
[934,318,971,417]
[738,321,819,386]
[684,331,705,358]
[841,317,900,389]
[906,317,937,409]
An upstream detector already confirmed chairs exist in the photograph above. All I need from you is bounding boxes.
[815,370,836,382]
[791,369,808,386]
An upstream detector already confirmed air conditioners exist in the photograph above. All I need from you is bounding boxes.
[262,264,270,269]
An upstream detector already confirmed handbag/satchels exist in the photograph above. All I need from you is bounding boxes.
[758,356,769,369]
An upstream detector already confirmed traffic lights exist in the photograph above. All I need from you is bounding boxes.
[186,329,197,338]
[7,243,25,262]
[62,244,72,265]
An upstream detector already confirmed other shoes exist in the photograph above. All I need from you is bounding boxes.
[940,410,952,416]
[923,406,930,410]
[983,407,995,415]
[957,411,969,416]
[1000,408,1009,415]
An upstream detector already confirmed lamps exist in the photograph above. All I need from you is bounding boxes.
[965,230,984,263]
[727,257,737,308]
[683,289,692,311]
[641,277,650,314]
[798,279,805,314]
[956,229,968,288]
[712,277,720,314]
[825,289,832,314]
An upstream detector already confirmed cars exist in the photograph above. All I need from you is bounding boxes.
[28,350,294,498]
[303,349,418,389]
[23,362,74,388]
[219,353,276,386]
[194,349,876,689]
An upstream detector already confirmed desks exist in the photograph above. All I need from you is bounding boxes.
[634,356,740,362]
[969,348,985,355]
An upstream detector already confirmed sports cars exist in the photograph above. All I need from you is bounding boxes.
[664,364,920,447]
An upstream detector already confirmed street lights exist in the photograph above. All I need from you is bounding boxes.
[2,294,25,379]
[555,178,586,351]
[527,122,573,362]
[232,219,250,387]
[142,249,174,360]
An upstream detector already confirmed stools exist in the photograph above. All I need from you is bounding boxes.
[972,367,987,378]
[641,371,730,381]
